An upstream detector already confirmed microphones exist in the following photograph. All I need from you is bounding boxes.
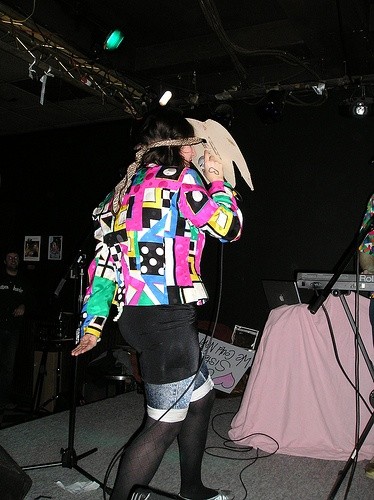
[197,156,228,183]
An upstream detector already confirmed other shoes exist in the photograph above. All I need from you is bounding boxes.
[179,489,235,500]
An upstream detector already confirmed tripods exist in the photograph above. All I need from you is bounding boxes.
[20,247,115,497]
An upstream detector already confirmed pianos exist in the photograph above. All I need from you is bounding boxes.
[297,271,374,500]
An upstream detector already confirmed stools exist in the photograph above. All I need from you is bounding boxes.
[81,366,125,405]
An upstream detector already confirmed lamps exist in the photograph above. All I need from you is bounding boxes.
[337,97,369,120]
[258,90,284,116]
[88,18,125,64]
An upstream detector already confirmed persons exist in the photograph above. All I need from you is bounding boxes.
[358,192,374,348]
[72,111,243,500]
[0,249,36,409]
